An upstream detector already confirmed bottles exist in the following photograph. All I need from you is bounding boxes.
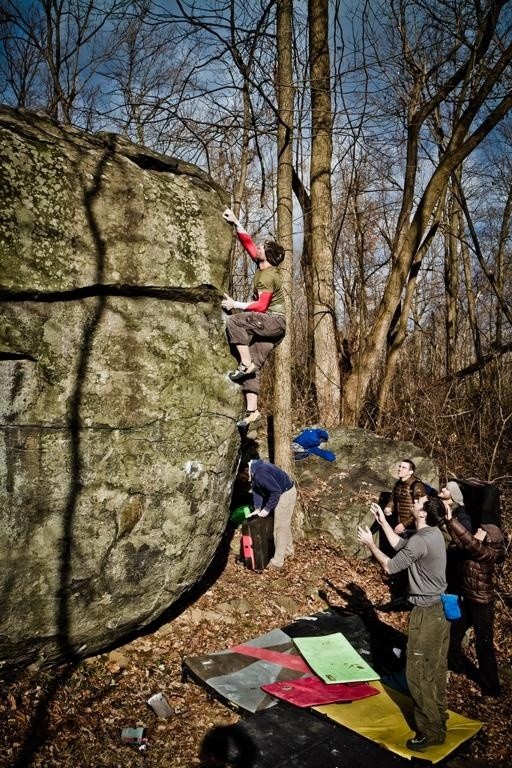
[446,481,464,508]
[480,523,504,545]
[264,237,286,267]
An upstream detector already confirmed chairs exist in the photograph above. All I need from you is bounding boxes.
[228,360,257,382]
[405,723,447,750]
[478,693,499,705]
[236,409,262,427]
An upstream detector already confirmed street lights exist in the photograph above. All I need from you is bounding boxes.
[440,593,462,620]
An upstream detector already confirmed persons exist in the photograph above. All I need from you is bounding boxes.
[237,458,297,574]
[355,492,451,749]
[436,479,470,587]
[443,504,505,705]
[220,206,289,431]
[381,458,428,542]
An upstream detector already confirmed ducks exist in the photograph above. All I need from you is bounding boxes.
[241,514,276,572]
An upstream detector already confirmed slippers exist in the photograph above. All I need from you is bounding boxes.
[409,479,438,498]
